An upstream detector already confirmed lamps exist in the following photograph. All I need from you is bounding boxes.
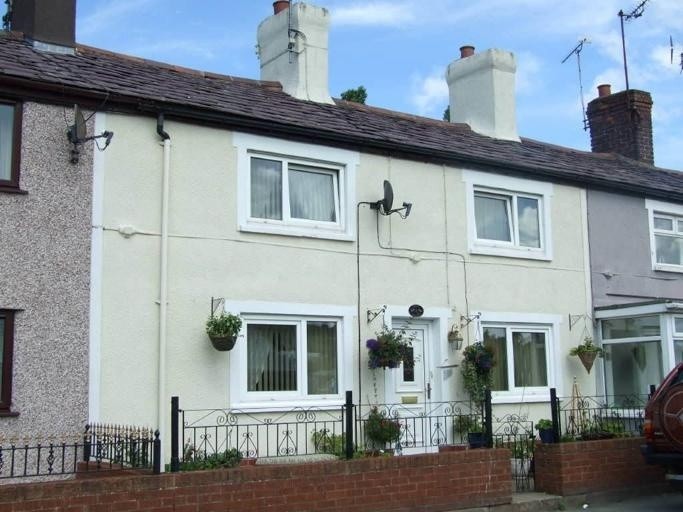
[447,324,463,350]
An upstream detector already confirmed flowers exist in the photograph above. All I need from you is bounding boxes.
[364,406,402,446]
[367,324,417,369]
[206,312,242,337]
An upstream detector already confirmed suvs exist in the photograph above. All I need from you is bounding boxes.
[642,361,682,482]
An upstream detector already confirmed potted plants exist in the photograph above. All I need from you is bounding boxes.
[454,413,486,448]
[570,337,606,373]
[535,419,555,443]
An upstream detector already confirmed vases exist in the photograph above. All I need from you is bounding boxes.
[390,360,400,368]
[208,337,237,351]
[374,447,395,458]
